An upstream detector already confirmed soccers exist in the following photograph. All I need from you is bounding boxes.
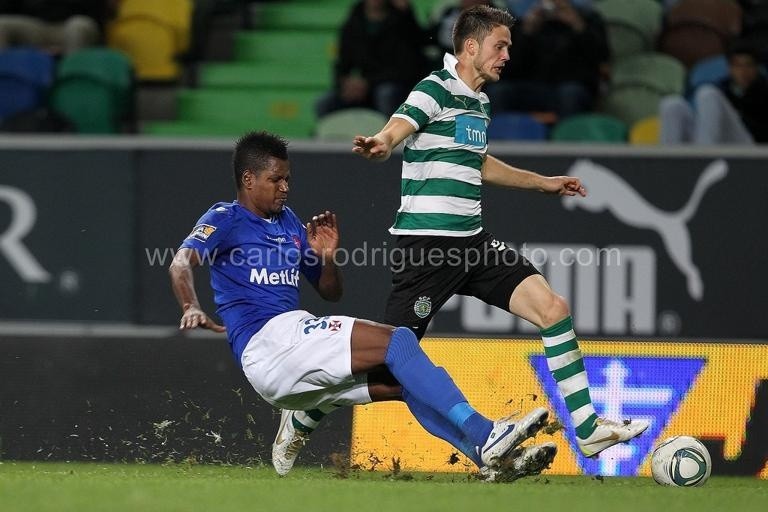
[651,436,712,487]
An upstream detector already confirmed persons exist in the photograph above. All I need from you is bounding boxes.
[168,129,559,483]
[658,44,768,144]
[271,4,651,477]
[1,1,121,57]
[313,0,614,133]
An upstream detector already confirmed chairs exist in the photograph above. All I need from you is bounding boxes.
[0,0,207,134]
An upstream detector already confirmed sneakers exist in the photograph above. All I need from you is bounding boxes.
[474,407,549,468]
[271,409,311,477]
[481,442,558,485]
[576,416,649,458]
